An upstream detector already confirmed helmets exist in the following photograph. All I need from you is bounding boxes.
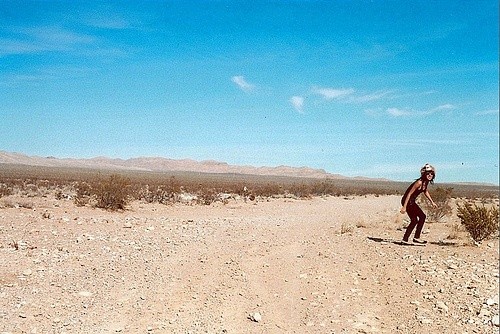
[421,163,435,173]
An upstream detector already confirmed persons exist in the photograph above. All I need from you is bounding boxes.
[400,163,438,246]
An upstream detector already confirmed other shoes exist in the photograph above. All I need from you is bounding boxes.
[413,238,427,244]
